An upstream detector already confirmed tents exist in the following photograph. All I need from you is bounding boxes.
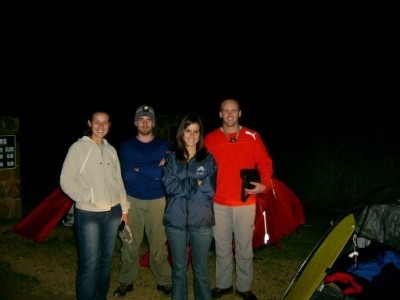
[281,188,400,300]
[12,170,305,266]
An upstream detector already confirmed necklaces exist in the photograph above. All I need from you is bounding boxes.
[223,123,240,142]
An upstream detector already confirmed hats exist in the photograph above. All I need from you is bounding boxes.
[134,105,156,121]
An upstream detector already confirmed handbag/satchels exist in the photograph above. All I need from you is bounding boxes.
[240,169,261,201]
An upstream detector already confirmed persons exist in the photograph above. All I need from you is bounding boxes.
[60,109,129,300]
[112,104,173,296]
[203,98,273,300]
[161,116,218,300]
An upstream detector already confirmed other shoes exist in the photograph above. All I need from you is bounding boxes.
[212,285,235,300]
[156,284,172,296]
[114,283,133,298]
[235,290,257,300]
